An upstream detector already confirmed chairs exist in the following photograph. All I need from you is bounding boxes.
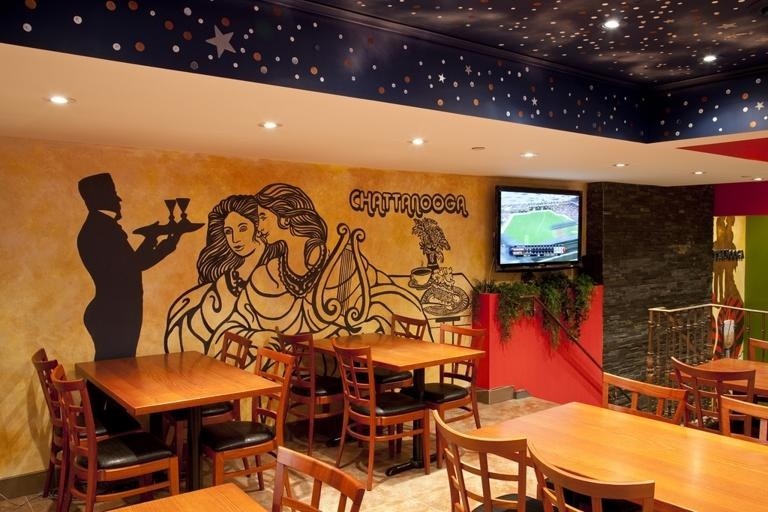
[50,364,182,512]
[600,372,688,431]
[401,323,489,471]
[269,447,365,512]
[196,346,298,489]
[526,437,656,512]
[357,313,426,452]
[273,327,344,459]
[718,395,768,441]
[31,348,145,511]
[670,355,757,441]
[328,336,433,493]
[747,336,768,364]
[157,332,253,471]
[432,407,558,512]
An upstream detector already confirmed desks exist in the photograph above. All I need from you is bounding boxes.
[667,357,768,398]
[295,332,486,477]
[460,400,767,512]
[75,350,283,494]
[111,481,279,512]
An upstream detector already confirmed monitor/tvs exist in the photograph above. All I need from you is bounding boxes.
[492,185,584,271]
